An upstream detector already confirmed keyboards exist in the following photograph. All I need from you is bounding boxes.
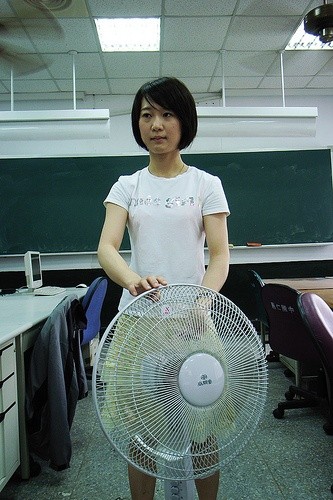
[34,286,66,296]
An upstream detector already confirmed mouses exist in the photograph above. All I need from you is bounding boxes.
[76,284,87,288]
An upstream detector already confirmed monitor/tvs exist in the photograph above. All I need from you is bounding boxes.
[23,251,42,294]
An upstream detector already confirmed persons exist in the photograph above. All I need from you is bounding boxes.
[98,75,229,500]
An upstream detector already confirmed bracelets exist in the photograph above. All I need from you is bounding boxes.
[194,291,214,300]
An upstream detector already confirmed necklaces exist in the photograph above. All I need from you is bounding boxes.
[176,163,185,177]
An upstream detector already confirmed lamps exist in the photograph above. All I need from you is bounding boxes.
[195,49,318,140]
[0,50,110,138]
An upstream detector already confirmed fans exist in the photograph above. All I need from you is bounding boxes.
[93,285,268,500]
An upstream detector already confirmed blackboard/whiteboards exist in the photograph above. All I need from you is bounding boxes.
[0,145,332,258]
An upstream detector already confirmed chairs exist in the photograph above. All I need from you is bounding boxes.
[26,275,112,470]
[244,269,333,435]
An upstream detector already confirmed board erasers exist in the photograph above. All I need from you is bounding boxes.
[247,242,261,246]
[229,244,233,247]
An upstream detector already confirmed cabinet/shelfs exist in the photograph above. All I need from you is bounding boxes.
[0,287,99,492]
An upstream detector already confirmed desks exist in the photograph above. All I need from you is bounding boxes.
[262,277,333,312]
